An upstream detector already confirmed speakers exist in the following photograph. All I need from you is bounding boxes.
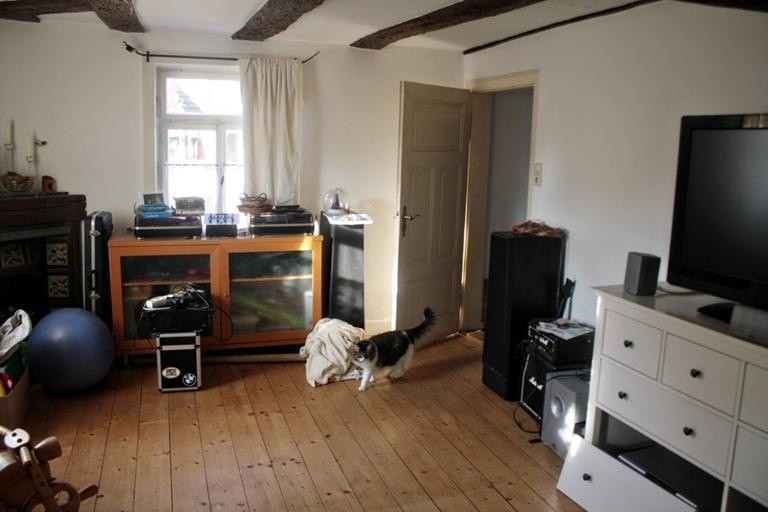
[520,346,590,421]
[481,232,565,402]
[622,251,661,297]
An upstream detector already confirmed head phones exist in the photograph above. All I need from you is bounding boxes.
[166,295,189,309]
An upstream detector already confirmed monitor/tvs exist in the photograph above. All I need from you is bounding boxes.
[666,114,768,328]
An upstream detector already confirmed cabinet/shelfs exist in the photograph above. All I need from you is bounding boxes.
[557,281,768,510]
[0,194,87,326]
[108,229,324,364]
[319,210,364,329]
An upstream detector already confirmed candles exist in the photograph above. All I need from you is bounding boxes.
[5,117,12,144]
[25,135,31,156]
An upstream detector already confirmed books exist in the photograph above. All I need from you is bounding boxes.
[536,317,593,340]
[322,212,374,225]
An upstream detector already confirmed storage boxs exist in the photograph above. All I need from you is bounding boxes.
[153,332,202,392]
[0,363,37,431]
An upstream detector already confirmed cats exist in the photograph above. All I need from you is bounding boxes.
[350,306,439,391]
